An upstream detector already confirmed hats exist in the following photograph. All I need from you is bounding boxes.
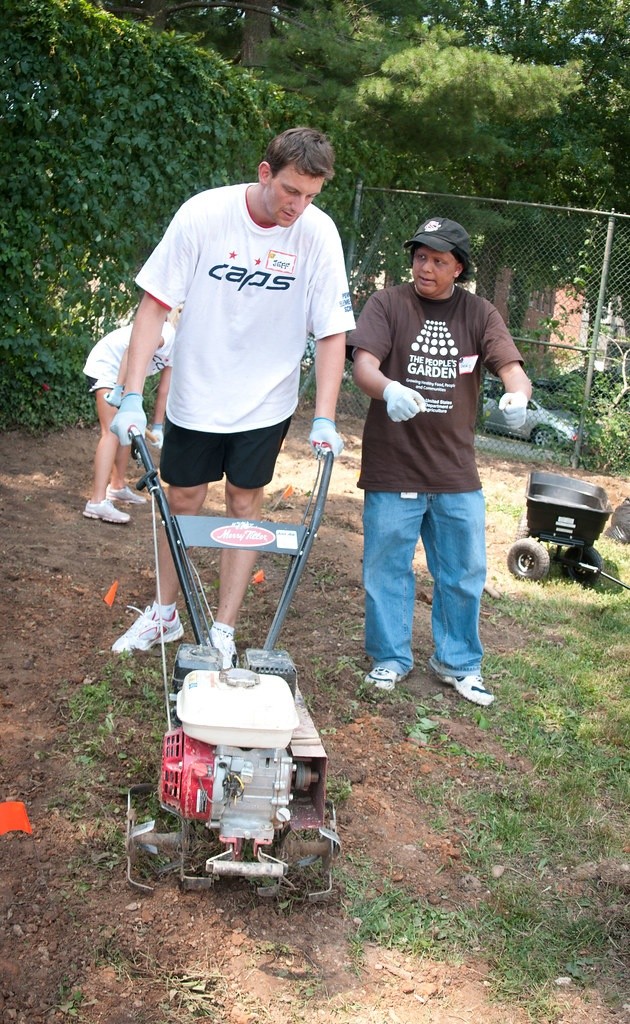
[403,217,471,257]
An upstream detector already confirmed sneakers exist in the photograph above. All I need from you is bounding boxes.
[112,605,185,655]
[82,499,130,523]
[106,485,146,504]
[363,661,414,691]
[200,627,239,669]
[429,654,494,706]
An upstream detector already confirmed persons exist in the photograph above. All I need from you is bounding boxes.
[80,308,177,524]
[342,216,533,707]
[104,123,355,667]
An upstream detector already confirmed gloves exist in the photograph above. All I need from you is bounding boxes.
[383,381,426,422]
[150,424,163,449]
[499,392,527,429]
[104,385,124,407]
[110,392,147,446]
[310,418,345,457]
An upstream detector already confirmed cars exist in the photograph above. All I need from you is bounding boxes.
[481,374,579,450]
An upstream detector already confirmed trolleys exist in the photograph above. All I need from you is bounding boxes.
[506,469,630,591]
